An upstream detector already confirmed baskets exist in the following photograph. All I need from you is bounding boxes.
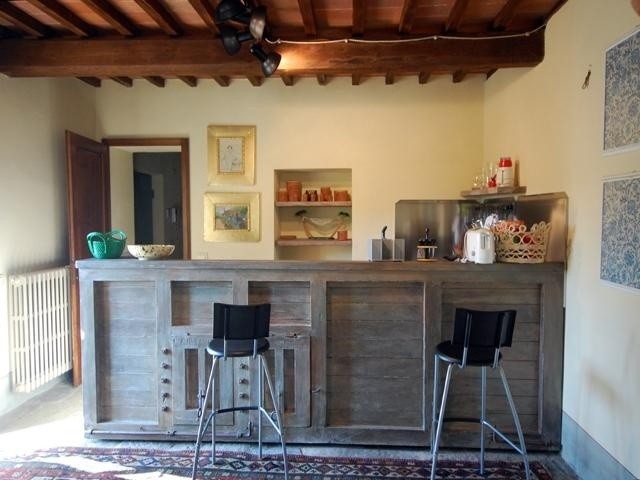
[490,221,552,265]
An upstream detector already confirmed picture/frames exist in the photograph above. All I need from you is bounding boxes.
[205,193,261,240]
[208,125,255,185]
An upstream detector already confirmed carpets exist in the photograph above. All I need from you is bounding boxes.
[0,446,554,480]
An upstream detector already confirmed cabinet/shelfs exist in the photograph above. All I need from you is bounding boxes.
[274,201,352,246]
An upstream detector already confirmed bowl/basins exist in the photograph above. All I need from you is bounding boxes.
[126,244,175,261]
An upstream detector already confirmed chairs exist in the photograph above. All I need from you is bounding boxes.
[192,303,289,480]
[431,307,531,480]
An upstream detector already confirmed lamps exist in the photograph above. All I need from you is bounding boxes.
[215,0,281,77]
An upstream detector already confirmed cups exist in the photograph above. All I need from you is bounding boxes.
[277,179,351,201]
[337,231,348,241]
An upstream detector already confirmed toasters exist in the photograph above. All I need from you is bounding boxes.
[463,226,497,265]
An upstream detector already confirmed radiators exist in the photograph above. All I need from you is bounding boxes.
[9,265,72,393]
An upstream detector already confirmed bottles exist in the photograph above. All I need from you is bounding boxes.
[471,156,515,188]
